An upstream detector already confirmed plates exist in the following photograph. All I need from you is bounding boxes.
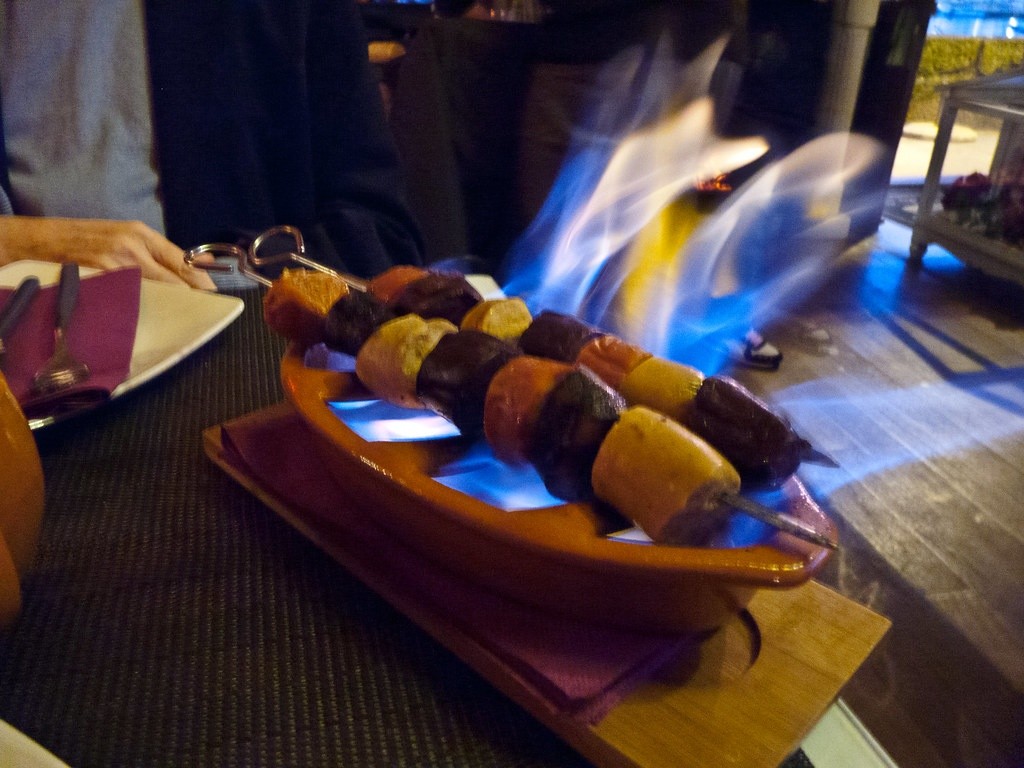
[0,259,244,429]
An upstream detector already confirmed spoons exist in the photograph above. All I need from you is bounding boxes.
[28,261,89,393]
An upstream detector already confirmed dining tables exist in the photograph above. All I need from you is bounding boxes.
[0,273,904,768]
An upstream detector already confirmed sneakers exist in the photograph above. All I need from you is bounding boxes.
[701,326,783,369]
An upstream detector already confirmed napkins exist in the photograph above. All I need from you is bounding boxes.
[0,264,144,423]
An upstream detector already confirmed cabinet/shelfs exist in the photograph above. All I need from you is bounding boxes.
[906,64,1024,288]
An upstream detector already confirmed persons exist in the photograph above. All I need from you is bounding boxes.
[1,1,435,289]
